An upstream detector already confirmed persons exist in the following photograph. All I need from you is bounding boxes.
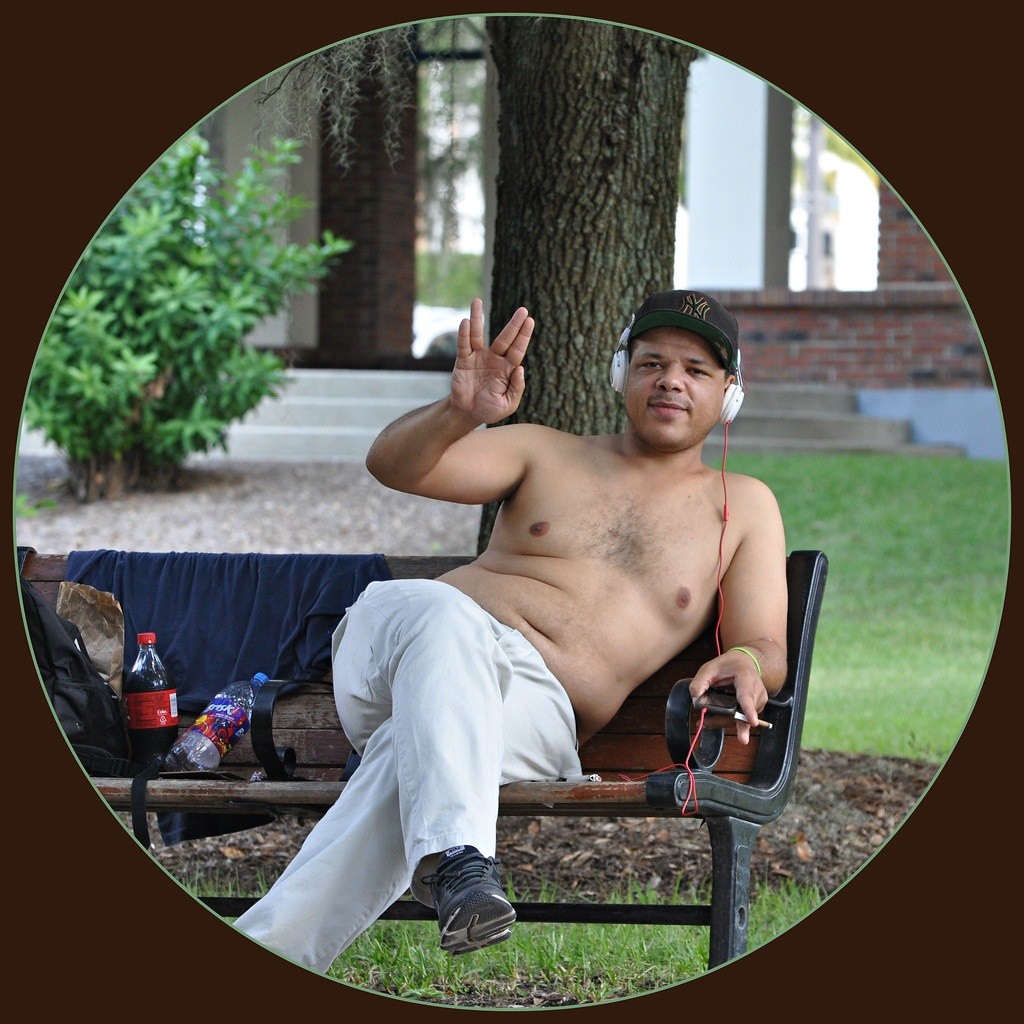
[225,288,789,975]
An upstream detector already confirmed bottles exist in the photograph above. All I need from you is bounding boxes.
[164,672,270,771]
[122,632,179,776]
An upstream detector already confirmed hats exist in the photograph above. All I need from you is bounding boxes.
[627,289,751,362]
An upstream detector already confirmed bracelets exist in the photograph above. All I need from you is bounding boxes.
[725,646,763,678]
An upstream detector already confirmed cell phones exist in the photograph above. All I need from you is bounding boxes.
[695,688,738,716]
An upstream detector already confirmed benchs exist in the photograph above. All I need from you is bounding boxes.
[10,547,829,979]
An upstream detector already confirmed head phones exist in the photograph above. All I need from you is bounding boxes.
[609,313,744,425]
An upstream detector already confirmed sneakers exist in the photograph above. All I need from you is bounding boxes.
[433,845,514,956]
